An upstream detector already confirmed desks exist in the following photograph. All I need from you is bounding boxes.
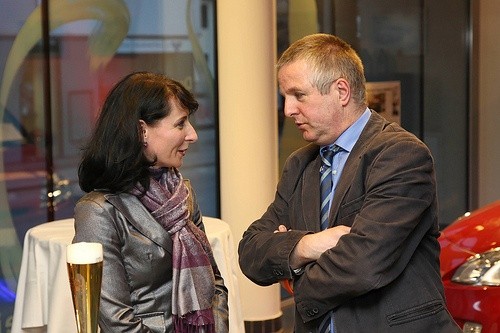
[11,215,245,333]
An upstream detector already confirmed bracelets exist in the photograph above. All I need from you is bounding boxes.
[284,278,296,296]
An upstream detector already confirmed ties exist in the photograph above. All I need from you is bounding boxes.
[319,144,344,333]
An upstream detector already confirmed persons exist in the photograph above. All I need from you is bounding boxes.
[238,33,460,333]
[74,71,229,333]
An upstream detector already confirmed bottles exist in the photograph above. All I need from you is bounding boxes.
[66,241,104,333]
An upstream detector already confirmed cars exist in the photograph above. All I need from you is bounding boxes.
[0,108,76,244]
[438,200,500,333]
